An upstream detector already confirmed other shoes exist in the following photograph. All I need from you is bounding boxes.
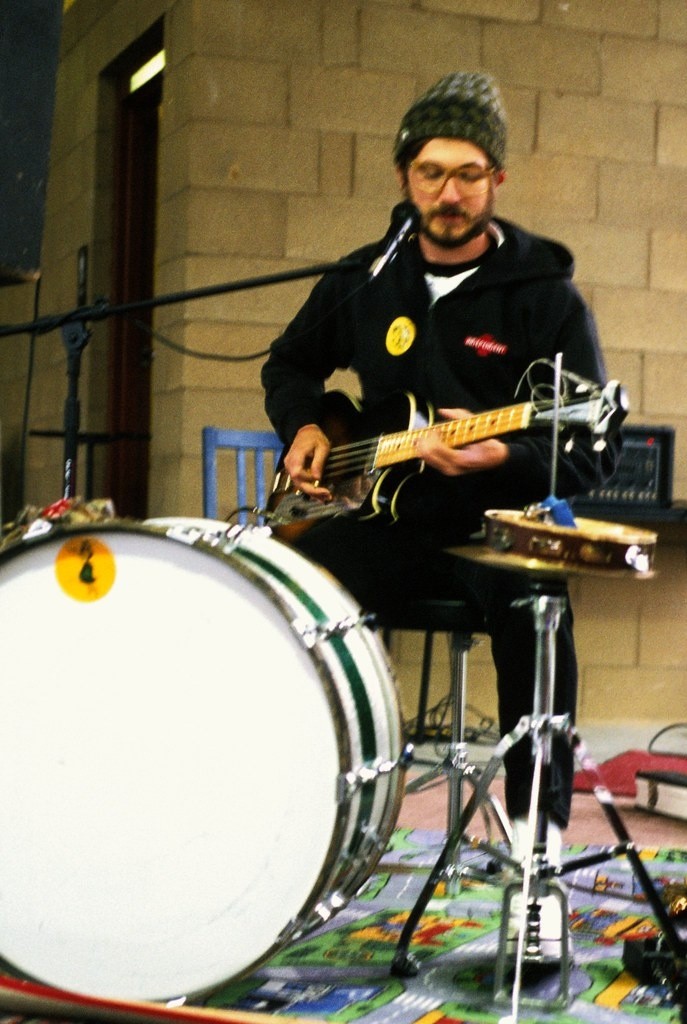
[505,818,571,965]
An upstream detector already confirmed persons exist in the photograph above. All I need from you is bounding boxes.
[259,68,625,963]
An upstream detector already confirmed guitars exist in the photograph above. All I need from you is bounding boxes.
[265,380,631,538]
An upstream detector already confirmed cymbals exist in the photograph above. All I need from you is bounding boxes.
[442,541,659,583]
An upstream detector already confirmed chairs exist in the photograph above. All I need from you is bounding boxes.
[202,427,285,532]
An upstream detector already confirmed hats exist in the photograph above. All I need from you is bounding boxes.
[392,70,508,170]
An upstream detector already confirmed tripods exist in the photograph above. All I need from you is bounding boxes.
[392,574,687,1024]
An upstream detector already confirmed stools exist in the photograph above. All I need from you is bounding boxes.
[389,582,517,898]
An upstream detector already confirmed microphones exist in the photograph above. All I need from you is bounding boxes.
[367,203,422,283]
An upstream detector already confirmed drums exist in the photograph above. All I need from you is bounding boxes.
[0,497,417,1006]
[484,500,660,574]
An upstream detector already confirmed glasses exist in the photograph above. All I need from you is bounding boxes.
[411,161,494,198]
